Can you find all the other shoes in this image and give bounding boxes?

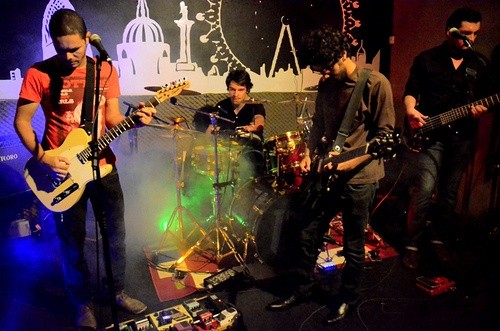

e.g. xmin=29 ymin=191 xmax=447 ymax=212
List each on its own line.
xmin=435 ymin=242 xmax=451 ymax=262
xmin=403 ymin=256 xmax=418 ymax=269
xmin=115 ymin=290 xmax=147 ymax=314
xmin=76 ymin=304 xmax=97 ymax=329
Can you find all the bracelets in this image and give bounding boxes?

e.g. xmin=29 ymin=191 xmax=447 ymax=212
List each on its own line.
xmin=39 ymin=153 xmax=45 ymax=162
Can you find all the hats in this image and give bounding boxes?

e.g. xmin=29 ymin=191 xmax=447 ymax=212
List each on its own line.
xmin=307 ymin=23 xmax=343 ymax=71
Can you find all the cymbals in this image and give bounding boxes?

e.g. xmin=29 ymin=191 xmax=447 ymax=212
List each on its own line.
xmin=278 ymin=98 xmax=316 ymax=103
xmin=144 ymin=87 xmax=201 ymax=95
xmin=145 ymin=124 xmax=201 ymax=133
xmin=236 ymin=99 xmax=272 ymax=104
xmin=158 ymin=134 xmax=192 ymax=138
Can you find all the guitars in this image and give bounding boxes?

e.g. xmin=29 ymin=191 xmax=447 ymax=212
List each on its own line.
xmin=24 ymin=77 xmax=191 ymax=212
xmin=301 ymin=132 xmax=407 ymax=212
xmin=402 ymin=94 xmax=500 ymax=153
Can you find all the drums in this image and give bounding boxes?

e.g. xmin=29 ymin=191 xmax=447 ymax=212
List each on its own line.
xmin=191 ymin=145 xmax=239 ymax=176
xmin=261 ymin=131 xmax=310 ymax=175
xmin=266 ymin=162 xmax=310 ymax=197
xmin=235 ymin=133 xmax=266 ymax=179
xmin=228 ymin=176 xmax=301 ymax=268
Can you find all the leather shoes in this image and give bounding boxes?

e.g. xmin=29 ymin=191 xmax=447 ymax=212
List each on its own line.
xmin=268 ymin=289 xmax=313 ymax=312
xmin=325 ymin=292 xmax=361 ymax=324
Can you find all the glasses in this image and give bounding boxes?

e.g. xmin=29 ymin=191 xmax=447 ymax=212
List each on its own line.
xmin=318 ymin=59 xmax=338 ymax=72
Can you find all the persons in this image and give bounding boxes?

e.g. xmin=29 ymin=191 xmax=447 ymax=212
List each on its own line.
xmin=14 ymin=9 xmax=157 ymax=329
xmin=267 ymin=25 xmax=395 ymax=312
xmin=403 ymin=7 xmax=500 ymax=269
xmin=208 ymin=70 xmax=266 ymax=176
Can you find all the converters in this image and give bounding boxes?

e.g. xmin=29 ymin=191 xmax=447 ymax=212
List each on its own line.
xmin=204 ymin=268 xmax=240 ymax=292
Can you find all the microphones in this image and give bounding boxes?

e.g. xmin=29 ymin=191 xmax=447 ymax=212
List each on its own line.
xmin=296 ymin=97 xmax=307 ymax=125
xmin=90 ymin=34 xmax=112 ymax=66
xmin=449 ymin=27 xmax=470 ymax=42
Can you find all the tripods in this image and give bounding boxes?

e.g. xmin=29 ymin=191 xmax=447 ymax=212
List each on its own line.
xmin=138 ymin=98 xmax=257 ymax=286
